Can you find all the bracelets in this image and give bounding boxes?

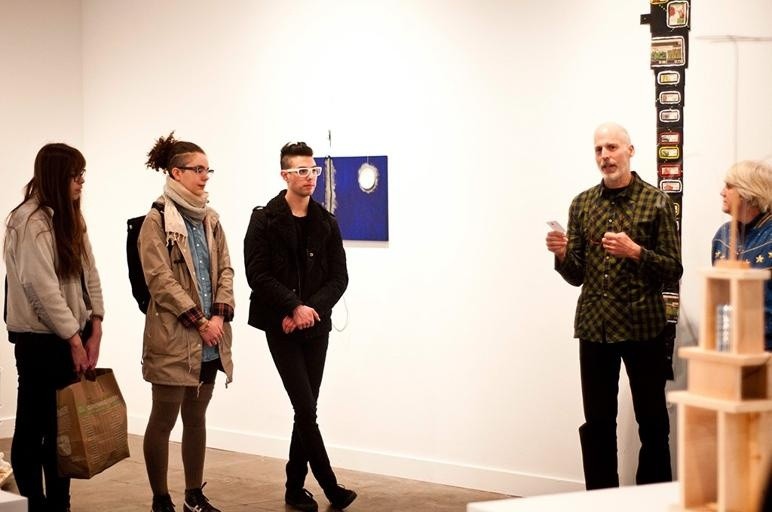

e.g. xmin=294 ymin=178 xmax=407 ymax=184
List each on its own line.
xmin=194 ymin=316 xmax=211 ymax=332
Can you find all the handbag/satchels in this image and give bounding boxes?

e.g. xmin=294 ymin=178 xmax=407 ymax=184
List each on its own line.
xmin=127 ymin=202 xmax=174 ymax=312
xmin=56 ymin=366 xmax=129 ymax=479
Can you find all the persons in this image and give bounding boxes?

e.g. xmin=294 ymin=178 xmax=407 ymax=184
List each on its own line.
xmin=3 ymin=142 xmax=104 ymax=512
xmin=137 ymin=129 xmax=235 ymax=512
xmin=546 ymin=121 xmax=684 ymax=490
xmin=711 ymin=161 xmax=772 ymax=353
xmin=244 ymin=142 xmax=357 ymax=512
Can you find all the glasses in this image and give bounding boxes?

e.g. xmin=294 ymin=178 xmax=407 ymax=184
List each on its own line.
xmin=72 ymin=169 xmax=86 ymax=183
xmin=288 ymin=167 xmax=322 ymax=177
xmin=177 ymin=165 xmax=214 ymax=178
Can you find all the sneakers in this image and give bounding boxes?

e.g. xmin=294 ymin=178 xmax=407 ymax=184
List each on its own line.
xmin=327 ymin=488 xmax=356 ymax=510
xmin=285 ymin=487 xmax=317 ymax=511
xmin=183 ymin=491 xmax=221 ymax=511
xmin=151 ymin=495 xmax=175 ymax=512
